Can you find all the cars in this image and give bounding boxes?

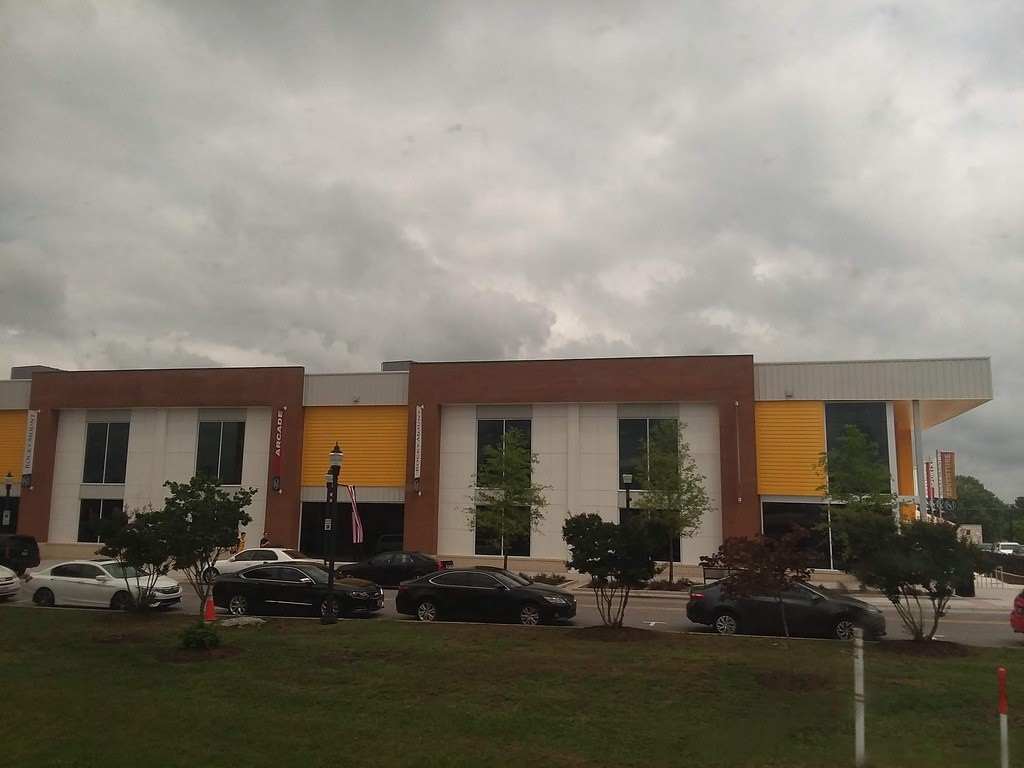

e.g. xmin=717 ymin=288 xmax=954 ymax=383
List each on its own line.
xmin=198 ymin=547 xmax=383 ymax=618
xmin=0 ymin=533 xmax=183 ymax=612
xmin=976 ymin=542 xmax=1024 ymax=559
xmin=395 ymin=566 xmax=577 ymax=624
xmin=336 ymin=550 xmax=454 ymax=589
xmin=685 ymin=570 xmax=888 ymax=642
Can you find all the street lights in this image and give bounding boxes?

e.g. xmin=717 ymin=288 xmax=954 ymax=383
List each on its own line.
xmin=622 ymin=472 xmax=632 ymax=520
xmin=4 ymin=469 xmax=12 ymax=534
xmin=321 ymin=441 xmax=345 ymax=622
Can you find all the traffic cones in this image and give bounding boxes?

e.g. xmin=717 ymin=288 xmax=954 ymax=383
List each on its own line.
xmin=203 ymin=582 xmax=217 ymax=621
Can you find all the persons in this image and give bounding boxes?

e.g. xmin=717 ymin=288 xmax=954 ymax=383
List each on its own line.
xmin=235 ymin=532 xmax=246 ymax=553
xmin=260 ymin=532 xmax=270 ymax=548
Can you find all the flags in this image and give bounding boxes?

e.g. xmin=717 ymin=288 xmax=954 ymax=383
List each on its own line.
xmin=347 ymin=485 xmax=363 ymax=543
